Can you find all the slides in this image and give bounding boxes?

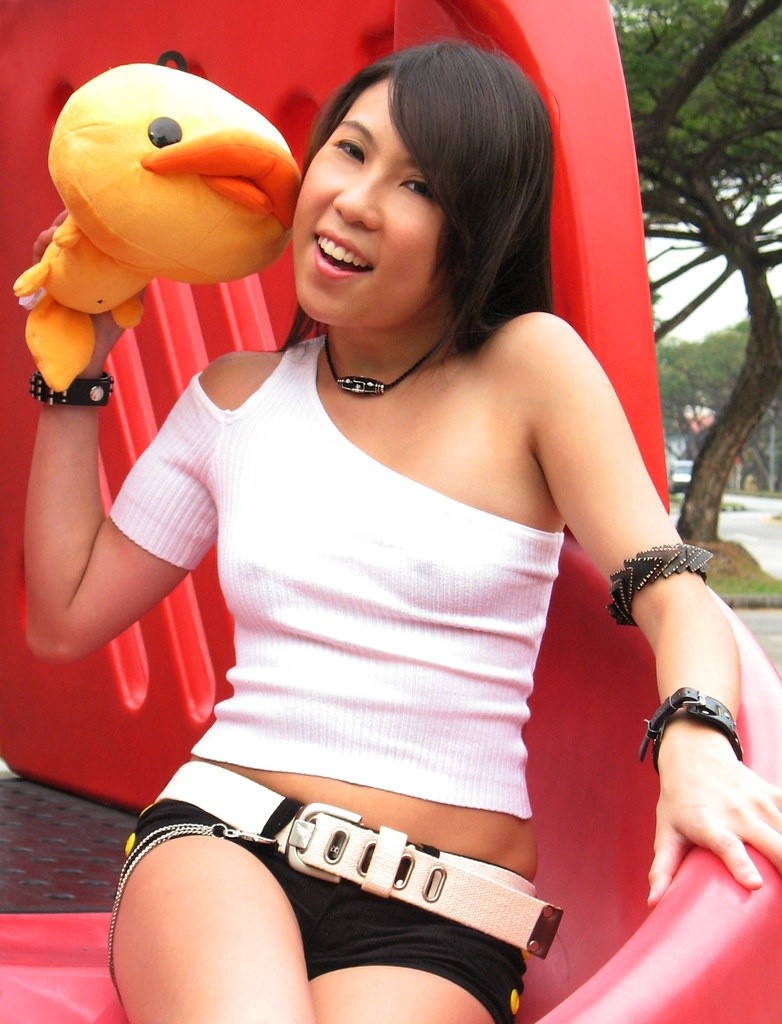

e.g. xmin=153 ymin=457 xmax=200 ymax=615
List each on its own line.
xmin=0 ymin=1 xmax=782 ymax=1024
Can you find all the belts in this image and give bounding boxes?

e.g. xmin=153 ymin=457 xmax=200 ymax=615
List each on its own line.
xmin=156 ymin=761 xmax=564 ymax=961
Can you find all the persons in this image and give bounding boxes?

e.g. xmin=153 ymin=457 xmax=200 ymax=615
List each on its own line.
xmin=25 ymin=42 xmax=780 ymax=1023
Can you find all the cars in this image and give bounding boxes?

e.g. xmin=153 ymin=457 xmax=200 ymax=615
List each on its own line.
xmin=669 ymin=460 xmax=693 ymax=493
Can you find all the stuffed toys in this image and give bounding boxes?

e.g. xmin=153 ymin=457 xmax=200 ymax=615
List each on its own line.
xmin=12 ymin=50 xmax=303 ymax=395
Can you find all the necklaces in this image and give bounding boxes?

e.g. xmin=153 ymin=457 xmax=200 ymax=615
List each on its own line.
xmin=324 ymin=317 xmax=453 ymax=397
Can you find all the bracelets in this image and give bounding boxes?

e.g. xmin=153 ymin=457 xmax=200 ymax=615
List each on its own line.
xmin=28 ymin=370 xmax=114 ymax=407
xmin=637 ymin=687 xmax=743 ymax=776
xmin=605 ymin=543 xmax=715 ymax=627
